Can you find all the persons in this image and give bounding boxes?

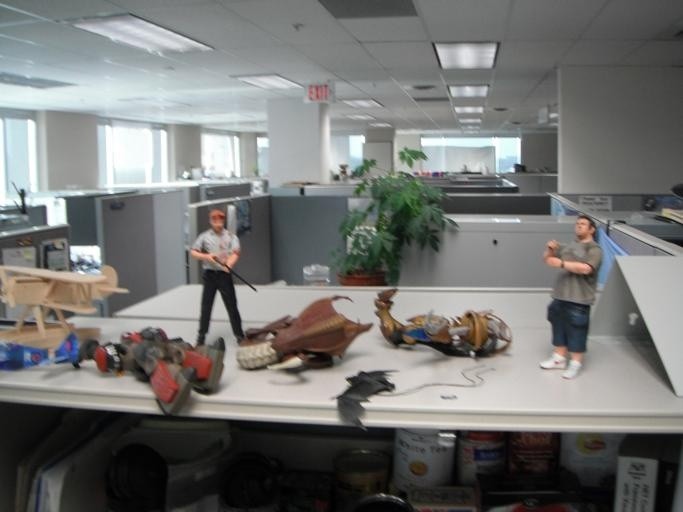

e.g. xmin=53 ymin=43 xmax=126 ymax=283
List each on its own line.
xmin=190 ymin=209 xmax=247 ymax=350
xmin=539 ymin=216 xmax=602 ymax=380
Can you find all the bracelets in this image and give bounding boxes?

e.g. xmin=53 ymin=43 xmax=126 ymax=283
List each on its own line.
xmin=560 ymin=260 xmax=564 ymax=269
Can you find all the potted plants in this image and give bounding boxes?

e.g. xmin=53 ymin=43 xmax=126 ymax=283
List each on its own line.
xmin=332 ymin=147 xmax=460 ymax=286
